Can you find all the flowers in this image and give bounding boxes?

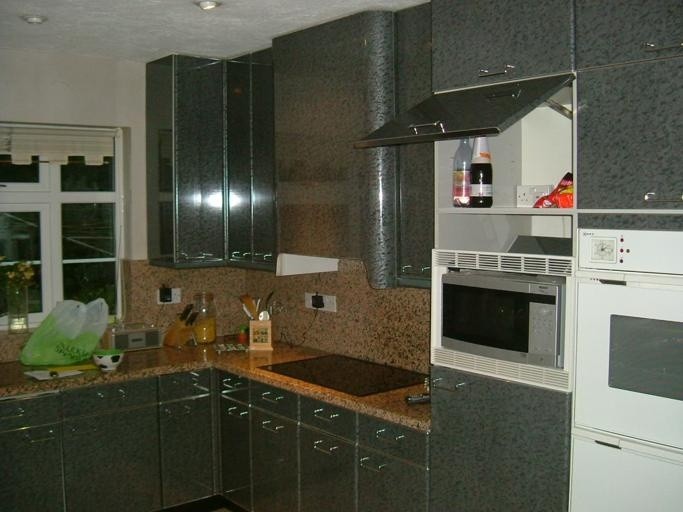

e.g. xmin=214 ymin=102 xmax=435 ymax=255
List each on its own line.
xmin=3 ymin=261 xmax=35 ymax=323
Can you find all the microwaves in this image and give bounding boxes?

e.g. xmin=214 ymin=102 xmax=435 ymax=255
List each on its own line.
xmin=439 ymin=267 xmax=567 ymax=370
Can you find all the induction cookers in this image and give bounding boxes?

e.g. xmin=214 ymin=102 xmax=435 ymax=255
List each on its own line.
xmin=256 ymin=354 xmax=430 ymax=397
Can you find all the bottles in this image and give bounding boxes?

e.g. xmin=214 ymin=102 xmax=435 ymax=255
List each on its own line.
xmin=191 ymin=291 xmax=216 ymax=343
xmin=452 ymin=135 xmax=493 ymax=208
xmin=237 ymin=324 xmax=249 ymax=346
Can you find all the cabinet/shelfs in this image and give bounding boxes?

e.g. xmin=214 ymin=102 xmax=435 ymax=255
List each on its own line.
xmin=162 ymin=369 xmax=215 ymax=512
xmin=220 ymin=371 xmax=428 ymax=511
xmin=0 ymin=378 xmax=160 ymax=512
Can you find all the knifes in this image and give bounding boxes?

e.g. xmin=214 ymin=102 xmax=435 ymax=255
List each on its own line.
xmin=179 ymin=304 xmax=200 ymax=327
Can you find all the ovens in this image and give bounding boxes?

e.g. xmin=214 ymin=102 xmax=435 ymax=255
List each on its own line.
xmin=568 ymin=228 xmax=683 ymax=512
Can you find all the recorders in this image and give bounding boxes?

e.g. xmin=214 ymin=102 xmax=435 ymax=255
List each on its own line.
xmin=111 ymin=321 xmax=161 ymax=353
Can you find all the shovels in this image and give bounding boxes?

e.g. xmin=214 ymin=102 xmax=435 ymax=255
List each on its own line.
xmin=240 ymin=290 xmax=273 ymax=320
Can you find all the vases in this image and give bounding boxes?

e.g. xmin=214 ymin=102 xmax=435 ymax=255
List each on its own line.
xmin=9 ymin=286 xmax=27 ymax=330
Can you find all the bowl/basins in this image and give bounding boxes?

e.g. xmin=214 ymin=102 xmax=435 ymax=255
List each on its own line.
xmin=93 ymin=349 xmax=124 ymax=372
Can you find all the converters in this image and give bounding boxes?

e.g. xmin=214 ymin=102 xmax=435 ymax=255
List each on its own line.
xmin=160 ymin=288 xmax=171 ymax=302
xmin=312 ymin=296 xmax=323 ymax=308
